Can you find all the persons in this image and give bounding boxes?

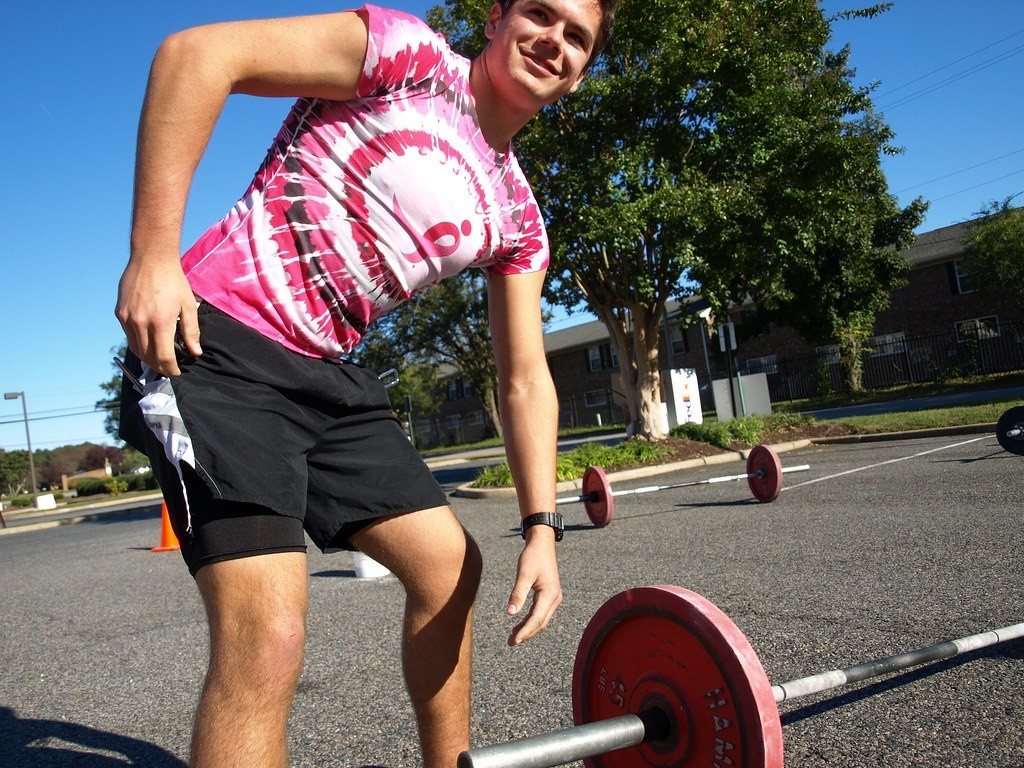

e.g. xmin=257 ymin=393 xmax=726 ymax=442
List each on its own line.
xmin=115 ymin=0 xmax=617 ymax=767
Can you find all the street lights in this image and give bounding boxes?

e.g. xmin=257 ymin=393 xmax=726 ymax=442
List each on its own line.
xmin=4 ymin=391 xmax=38 ymax=507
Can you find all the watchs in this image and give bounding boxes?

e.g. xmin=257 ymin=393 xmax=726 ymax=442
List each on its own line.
xmin=520 ymin=512 xmax=565 ymax=541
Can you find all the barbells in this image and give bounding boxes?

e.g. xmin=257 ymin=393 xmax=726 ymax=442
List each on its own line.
xmin=454 ymin=585 xmax=1024 ymax=767
xmin=555 ymin=444 xmax=811 ymax=528
xmin=996 ymin=406 xmax=1024 ymax=457
